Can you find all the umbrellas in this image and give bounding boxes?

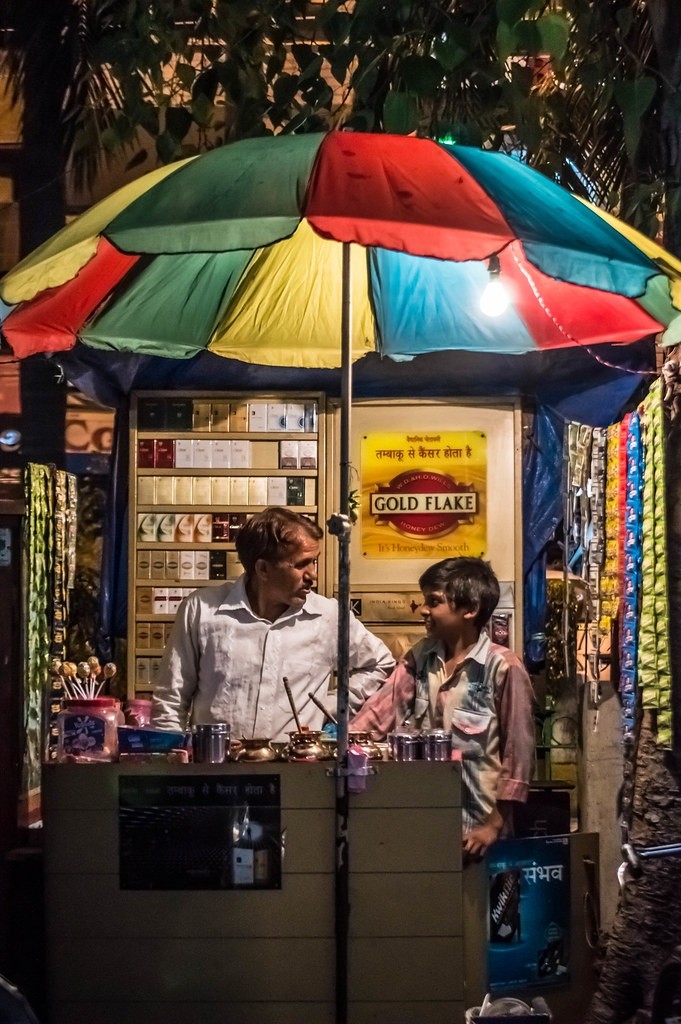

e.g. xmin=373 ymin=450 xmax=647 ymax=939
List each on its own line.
xmin=0 ymin=131 xmax=680 ymax=1024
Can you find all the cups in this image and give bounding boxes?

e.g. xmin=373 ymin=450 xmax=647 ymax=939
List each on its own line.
xmin=388 ymin=730 xmax=453 ymax=761
xmin=192 ymin=723 xmax=230 ymax=764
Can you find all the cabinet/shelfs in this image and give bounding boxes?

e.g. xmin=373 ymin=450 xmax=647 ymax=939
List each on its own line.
xmin=125 ymin=390 xmax=325 ymax=699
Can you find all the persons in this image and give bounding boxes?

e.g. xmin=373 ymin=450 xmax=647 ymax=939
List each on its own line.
xmin=349 ymin=557 xmax=534 ymax=863
xmin=149 ymin=505 xmax=394 ymax=749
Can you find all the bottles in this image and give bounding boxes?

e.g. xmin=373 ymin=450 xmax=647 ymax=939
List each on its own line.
xmin=122 ymin=699 xmax=151 ymax=727
xmin=57 ymin=698 xmax=125 ymax=763
